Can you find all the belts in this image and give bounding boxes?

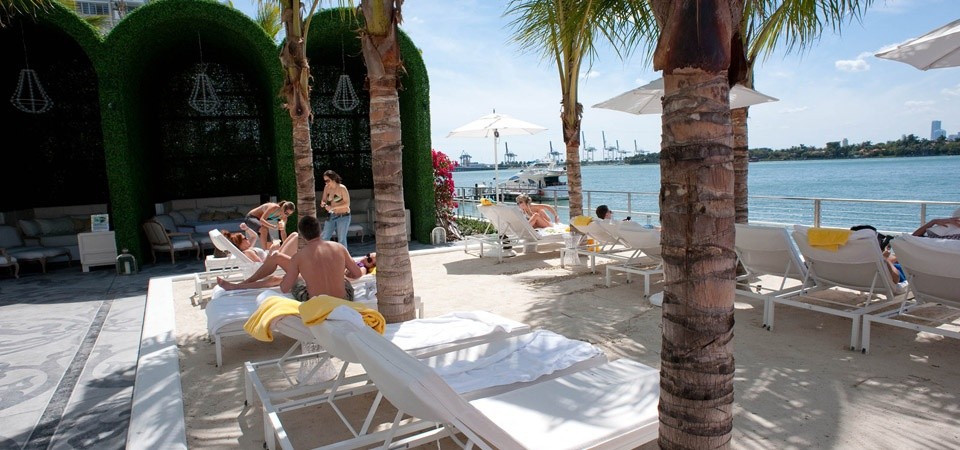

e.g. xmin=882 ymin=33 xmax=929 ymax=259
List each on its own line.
xmin=326 ymin=213 xmax=350 ymax=221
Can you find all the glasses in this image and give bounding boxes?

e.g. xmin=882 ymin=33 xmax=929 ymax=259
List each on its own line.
xmin=608 ymin=209 xmax=612 ymax=214
xmin=241 ymin=236 xmax=249 ymax=241
xmin=284 ymin=208 xmax=291 ymax=216
xmin=516 ymin=201 xmax=524 ymax=206
xmin=366 ymin=253 xmax=374 ymax=265
xmin=324 ymin=179 xmax=331 ymax=183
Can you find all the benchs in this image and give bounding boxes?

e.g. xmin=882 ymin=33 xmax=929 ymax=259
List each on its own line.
xmin=315 ymin=186 xmax=412 ymax=242
xmin=155 ymin=191 xmax=263 ymax=252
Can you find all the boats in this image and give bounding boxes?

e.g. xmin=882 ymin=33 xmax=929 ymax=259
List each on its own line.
xmin=494 ymin=162 xmax=570 ymax=200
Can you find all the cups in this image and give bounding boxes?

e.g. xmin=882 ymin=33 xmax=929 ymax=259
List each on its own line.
xmin=647 ymin=215 xmax=651 ymax=225
xmin=279 ymin=221 xmax=285 ymax=231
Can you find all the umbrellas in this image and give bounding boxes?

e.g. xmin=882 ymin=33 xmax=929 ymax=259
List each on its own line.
xmin=448 ymin=108 xmax=549 ymax=205
xmin=874 ymin=17 xmax=960 ymax=72
xmin=590 ymin=75 xmax=781 ymax=116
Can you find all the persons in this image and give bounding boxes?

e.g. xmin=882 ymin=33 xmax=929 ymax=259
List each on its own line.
xmin=595 ymin=204 xmax=661 ymax=230
xmin=849 ymin=209 xmax=960 ymax=285
xmin=209 ymin=164 xmax=378 ymax=313
xmin=515 ymin=192 xmax=559 ymax=230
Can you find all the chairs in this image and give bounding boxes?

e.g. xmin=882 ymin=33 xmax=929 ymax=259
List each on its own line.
xmin=478 ymin=201 xmax=540 ymax=258
xmin=259 ymin=298 xmax=622 ymax=448
xmin=1 ymin=203 xmax=110 ymax=279
xmin=497 ymin=201 xmax=572 ymax=257
xmin=193 ymin=224 xmax=432 ymax=368
xmin=573 ymin=212 xmax=958 ymax=361
xmin=239 ymin=296 xmax=534 ymax=450
xmin=143 ymin=220 xmax=201 ymax=264
xmin=338 ymin=318 xmax=659 ymax=449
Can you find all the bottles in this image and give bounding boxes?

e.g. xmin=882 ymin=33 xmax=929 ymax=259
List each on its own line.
xmin=324 ymin=204 xmax=335 ymax=215
